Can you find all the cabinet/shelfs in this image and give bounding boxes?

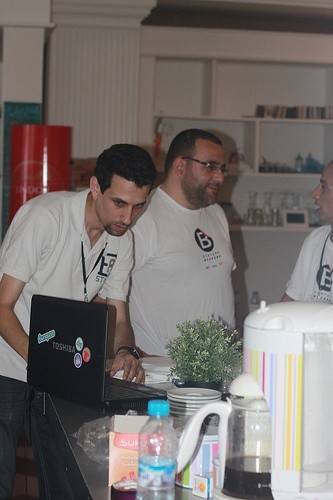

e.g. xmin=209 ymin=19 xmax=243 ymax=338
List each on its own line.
xmin=142 ymin=26 xmax=333 ymax=231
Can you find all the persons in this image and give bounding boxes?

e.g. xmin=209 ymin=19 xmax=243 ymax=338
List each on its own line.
xmin=280 ymin=160 xmax=333 ymax=305
xmin=0 ymin=143 xmax=157 ymax=500
xmin=90 ymin=127 xmax=237 ymax=355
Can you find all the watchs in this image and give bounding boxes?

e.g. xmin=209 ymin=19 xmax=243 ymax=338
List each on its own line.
xmin=114 ymin=346 xmax=140 ymax=358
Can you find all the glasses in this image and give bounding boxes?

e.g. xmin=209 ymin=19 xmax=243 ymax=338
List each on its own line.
xmin=183 ymin=157 xmax=228 ymax=176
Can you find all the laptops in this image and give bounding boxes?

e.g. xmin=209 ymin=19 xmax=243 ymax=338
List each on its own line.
xmin=28 ymin=294 xmax=168 ymax=411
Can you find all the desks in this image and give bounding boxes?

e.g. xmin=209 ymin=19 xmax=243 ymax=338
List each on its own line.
xmin=29 ymin=386 xmax=146 ymax=500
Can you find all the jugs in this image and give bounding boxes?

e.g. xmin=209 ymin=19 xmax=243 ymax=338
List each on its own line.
xmin=175 ymin=374 xmax=274 ymax=500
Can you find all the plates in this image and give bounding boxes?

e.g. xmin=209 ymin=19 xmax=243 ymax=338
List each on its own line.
xmin=166 ymin=388 xmax=222 ymax=415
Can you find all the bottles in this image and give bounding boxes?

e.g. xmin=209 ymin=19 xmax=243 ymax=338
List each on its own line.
xmin=259 ymin=192 xmax=274 ymax=226
xmin=277 ymin=193 xmax=285 ymax=225
xmin=136 ymin=400 xmax=176 ymax=500
xmin=296 ymin=154 xmax=303 ymax=173
xmin=244 ymin=191 xmax=258 ymax=226
xmin=251 ymin=291 xmax=260 ymax=311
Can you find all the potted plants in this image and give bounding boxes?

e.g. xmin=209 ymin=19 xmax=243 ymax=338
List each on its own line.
xmin=165 ymin=315 xmax=243 ymax=391
